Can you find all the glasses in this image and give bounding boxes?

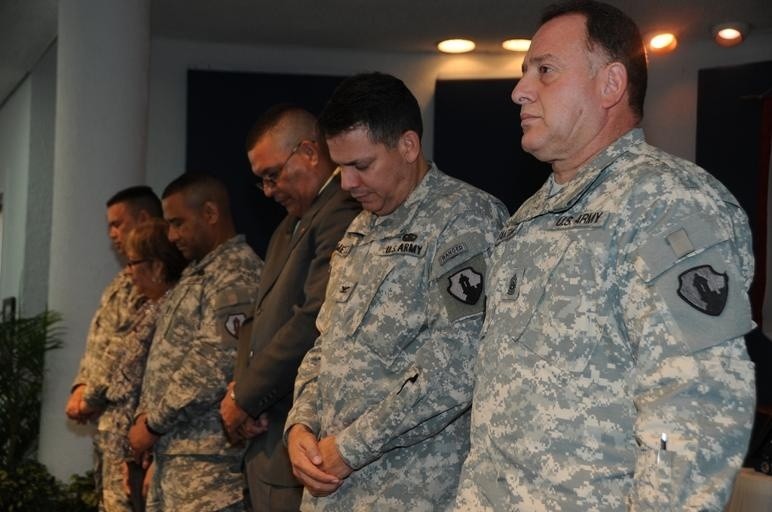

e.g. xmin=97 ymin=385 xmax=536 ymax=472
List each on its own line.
xmin=257 ymin=140 xmax=316 ymax=190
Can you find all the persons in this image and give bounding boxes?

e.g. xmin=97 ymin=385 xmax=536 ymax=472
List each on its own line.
xmin=284 ymin=68 xmax=509 ymax=512
xmin=65 ymin=186 xmax=191 ymax=512
xmin=452 ymin=6 xmax=757 ymax=512
xmin=126 ymin=170 xmax=265 ymax=512
xmin=217 ymin=105 xmax=362 ymax=512
